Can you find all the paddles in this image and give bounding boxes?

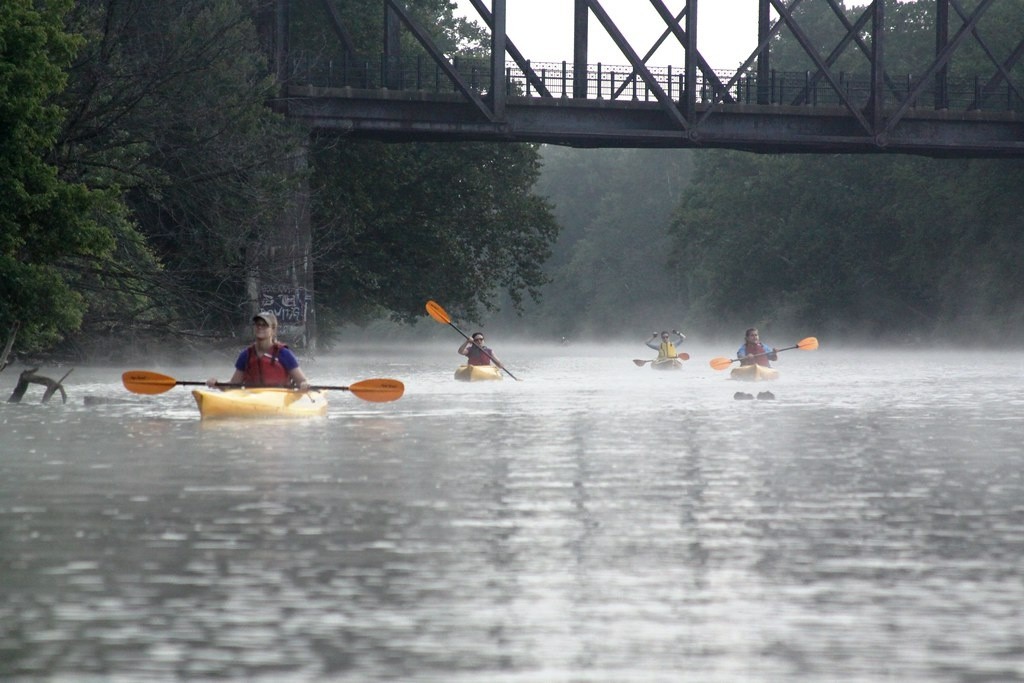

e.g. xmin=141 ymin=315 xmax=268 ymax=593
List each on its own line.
xmin=633 ymin=352 xmax=690 ymax=367
xmin=710 ymin=336 xmax=818 ymax=371
xmin=425 ymin=299 xmax=519 ymax=380
xmin=121 ymin=371 xmax=405 ymax=403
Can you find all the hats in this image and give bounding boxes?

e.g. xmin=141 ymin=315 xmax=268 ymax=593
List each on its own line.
xmin=252 ymin=312 xmax=277 ymax=328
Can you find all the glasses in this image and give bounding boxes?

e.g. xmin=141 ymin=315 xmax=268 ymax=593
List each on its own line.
xmin=474 ymin=337 xmax=484 ymax=342
xmin=255 ymin=323 xmax=269 ymax=330
xmin=662 ymin=336 xmax=668 ymax=339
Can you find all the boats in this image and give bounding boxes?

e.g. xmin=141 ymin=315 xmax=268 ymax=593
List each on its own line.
xmin=730 ymin=364 xmax=778 ymax=382
xmin=454 ymin=363 xmax=505 ymax=382
xmin=650 ymin=357 xmax=684 ymax=370
xmin=189 ymin=387 xmax=330 ymax=419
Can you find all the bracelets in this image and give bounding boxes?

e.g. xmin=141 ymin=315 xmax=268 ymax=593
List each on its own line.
xmin=678 ymin=332 xmax=680 ymax=335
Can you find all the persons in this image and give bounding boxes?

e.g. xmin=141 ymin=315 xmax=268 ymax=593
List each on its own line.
xmin=205 ymin=311 xmax=311 ymax=390
xmin=458 ymin=332 xmax=505 ymax=368
xmin=736 ymin=327 xmax=779 ymax=368
xmin=644 ymin=329 xmax=686 ymax=361
